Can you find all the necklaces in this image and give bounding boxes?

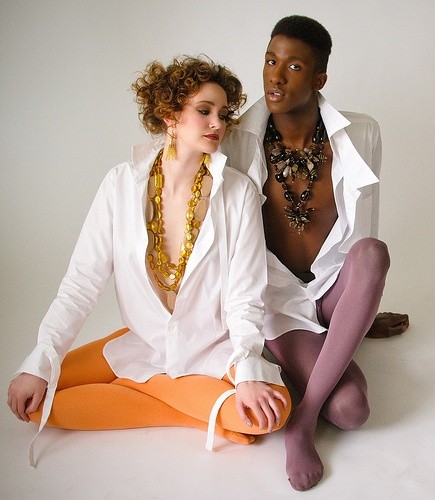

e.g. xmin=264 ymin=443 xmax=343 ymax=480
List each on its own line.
xmin=145 ymin=149 xmax=211 ymax=311
xmin=263 ymin=107 xmax=327 ymax=237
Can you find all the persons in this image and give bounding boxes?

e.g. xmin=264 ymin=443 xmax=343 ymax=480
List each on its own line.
xmin=6 ymin=54 xmax=292 ymax=468
xmin=216 ymin=15 xmax=411 ymax=492
